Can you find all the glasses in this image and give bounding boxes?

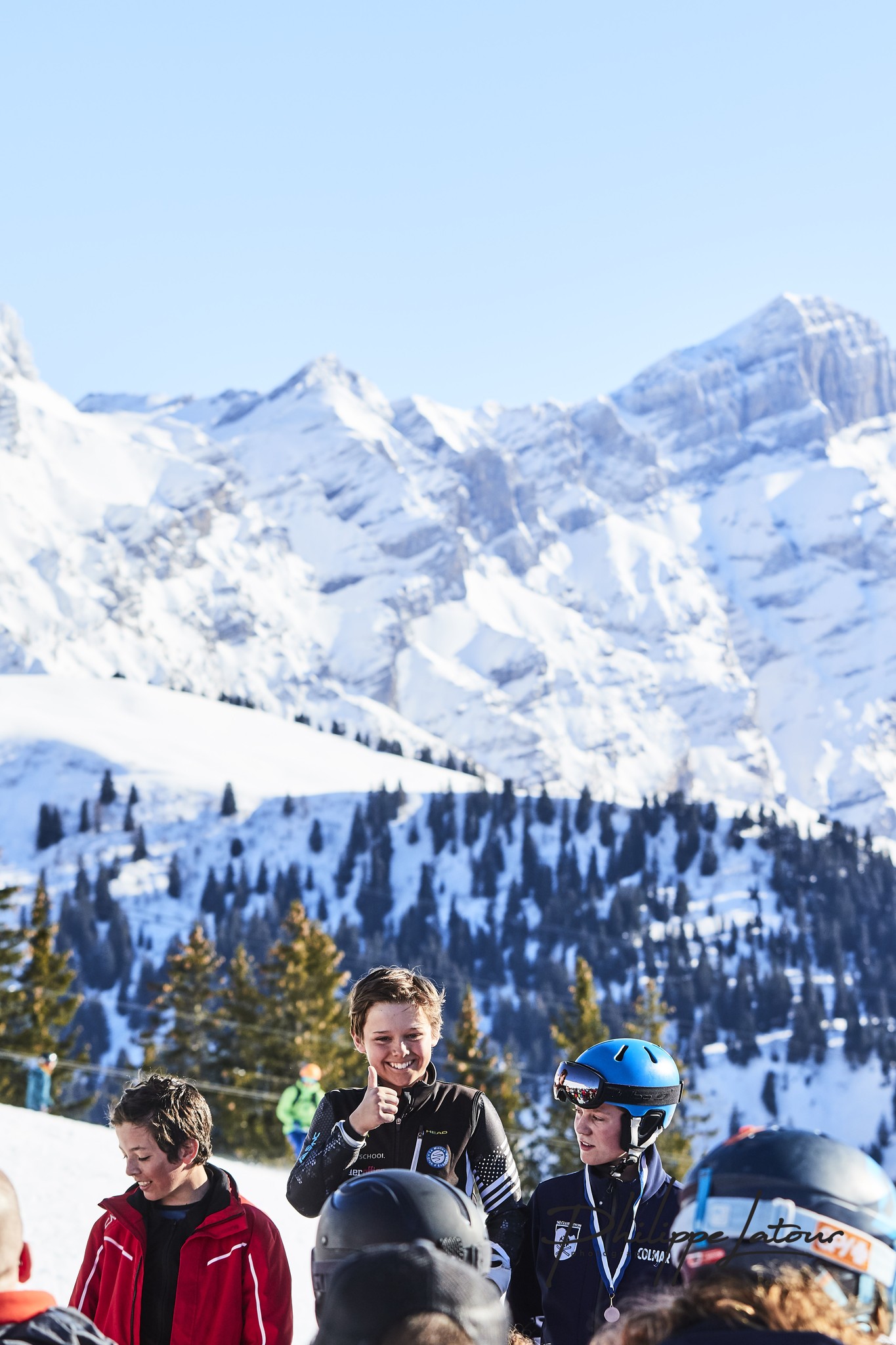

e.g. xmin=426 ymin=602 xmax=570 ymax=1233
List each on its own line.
xmin=553 ymin=1059 xmax=609 ymax=1110
xmin=301 ymin=1077 xmax=318 ymax=1084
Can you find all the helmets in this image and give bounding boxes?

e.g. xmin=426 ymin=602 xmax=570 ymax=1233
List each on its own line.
xmin=666 ymin=1123 xmax=896 ymax=1321
xmin=312 ymin=1168 xmax=487 ymax=1317
xmin=298 ymin=1063 xmax=321 ymax=1082
xmin=573 ymin=1036 xmax=681 ymax=1148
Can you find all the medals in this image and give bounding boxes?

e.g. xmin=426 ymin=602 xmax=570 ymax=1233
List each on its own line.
xmin=603 ymin=1305 xmax=620 ymax=1322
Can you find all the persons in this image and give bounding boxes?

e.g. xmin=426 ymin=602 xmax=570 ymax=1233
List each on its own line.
xmin=25 ymin=1050 xmax=60 ymax=1115
xmin=287 ymin=965 xmax=540 ymax=1300
xmin=310 ymin=1242 xmax=513 ymax=1345
xmin=591 ymin=1127 xmax=895 ymax=1345
xmin=509 ymin=1035 xmax=692 ymax=1344
xmin=276 ymin=1061 xmax=326 ymax=1161
xmin=0 ymin=1166 xmax=121 ymax=1345
xmin=312 ymin=1166 xmax=494 ymax=1328
xmin=550 ymin=1067 xmax=597 ymax=1104
xmin=67 ymin=1070 xmax=294 ymax=1344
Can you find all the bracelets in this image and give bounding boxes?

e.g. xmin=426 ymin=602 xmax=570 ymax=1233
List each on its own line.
xmin=344 ymin=1117 xmax=368 ymax=1142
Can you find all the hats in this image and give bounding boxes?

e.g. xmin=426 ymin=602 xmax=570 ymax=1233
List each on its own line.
xmin=316 ymin=1244 xmax=514 ymax=1345
